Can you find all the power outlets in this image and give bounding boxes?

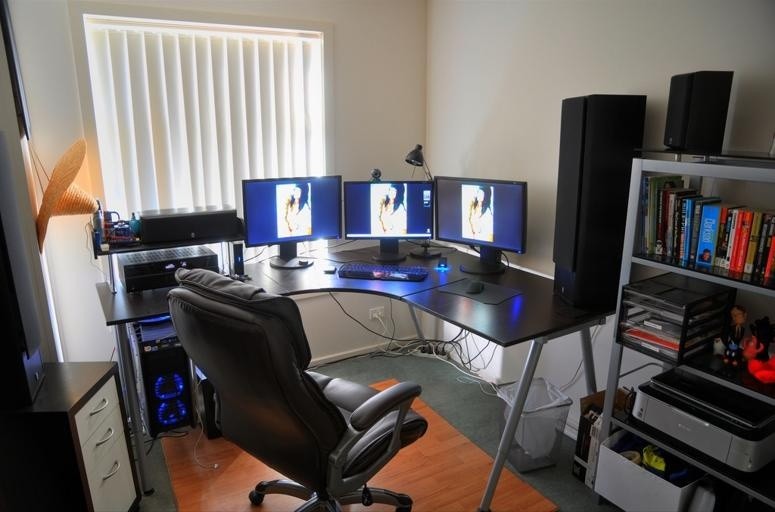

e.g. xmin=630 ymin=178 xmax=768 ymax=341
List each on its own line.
xmin=368 ymin=306 xmax=385 ymax=321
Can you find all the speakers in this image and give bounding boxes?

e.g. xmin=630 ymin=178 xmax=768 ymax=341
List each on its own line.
xmin=229 ymin=241 xmax=245 ymax=278
xmin=663 ymin=71 xmax=735 ymax=154
xmin=552 ymin=94 xmax=649 ymax=314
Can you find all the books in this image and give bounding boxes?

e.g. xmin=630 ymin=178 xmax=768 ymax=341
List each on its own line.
xmin=619 ymin=288 xmax=729 ymax=360
xmin=639 ymin=172 xmax=775 ymax=281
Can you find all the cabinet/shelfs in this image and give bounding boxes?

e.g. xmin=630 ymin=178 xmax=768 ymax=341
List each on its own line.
xmin=28 ymin=360 xmax=143 ymax=512
xmin=590 ymin=154 xmax=775 ymax=512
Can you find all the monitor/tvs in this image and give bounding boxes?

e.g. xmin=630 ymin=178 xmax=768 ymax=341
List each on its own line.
xmin=241 ymin=174 xmax=342 ymax=270
xmin=343 ymin=180 xmax=434 ymax=262
xmin=434 ymin=174 xmax=528 ymax=276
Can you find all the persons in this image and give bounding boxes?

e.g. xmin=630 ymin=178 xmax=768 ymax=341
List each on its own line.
xmin=467 ymin=186 xmax=493 ymax=241
xmin=280 ymin=181 xmax=311 ymax=235
xmin=375 ymin=183 xmax=408 ymax=234
xmin=712 ymin=301 xmax=775 ymax=384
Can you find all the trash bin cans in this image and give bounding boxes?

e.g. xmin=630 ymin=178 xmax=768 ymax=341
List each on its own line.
xmin=497 ymin=377 xmax=573 ymax=474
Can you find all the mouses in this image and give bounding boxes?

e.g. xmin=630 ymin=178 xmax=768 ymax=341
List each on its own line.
xmin=466 ymin=280 xmax=485 ymax=295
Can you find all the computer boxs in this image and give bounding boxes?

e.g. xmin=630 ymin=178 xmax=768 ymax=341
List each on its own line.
xmin=126 ymin=315 xmax=193 ymax=438
xmin=192 ymin=364 xmax=221 ymax=441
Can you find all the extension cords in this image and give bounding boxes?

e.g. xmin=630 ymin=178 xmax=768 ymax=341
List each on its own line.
xmin=408 ymin=349 xmax=449 ymax=361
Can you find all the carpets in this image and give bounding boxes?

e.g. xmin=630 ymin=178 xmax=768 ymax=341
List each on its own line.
xmin=159 ymin=378 xmax=562 ymax=512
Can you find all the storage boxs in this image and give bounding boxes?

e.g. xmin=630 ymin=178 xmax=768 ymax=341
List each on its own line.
xmin=593 ymin=428 xmax=712 ymax=512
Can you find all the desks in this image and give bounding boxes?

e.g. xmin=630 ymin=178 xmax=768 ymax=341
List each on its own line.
xmin=94 ymin=237 xmax=618 ymax=512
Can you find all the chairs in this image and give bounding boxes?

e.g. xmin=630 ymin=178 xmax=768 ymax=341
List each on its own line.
xmin=165 ymin=266 xmax=428 ymax=512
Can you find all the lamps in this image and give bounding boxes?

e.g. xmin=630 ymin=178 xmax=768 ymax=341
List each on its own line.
xmin=404 ymin=144 xmax=433 ymax=182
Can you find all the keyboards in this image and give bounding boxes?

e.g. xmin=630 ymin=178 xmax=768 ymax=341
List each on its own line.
xmin=337 ymin=261 xmax=429 ymax=283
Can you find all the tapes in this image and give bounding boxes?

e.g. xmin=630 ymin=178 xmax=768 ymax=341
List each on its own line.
xmin=620 ymin=451 xmax=641 ymax=464
